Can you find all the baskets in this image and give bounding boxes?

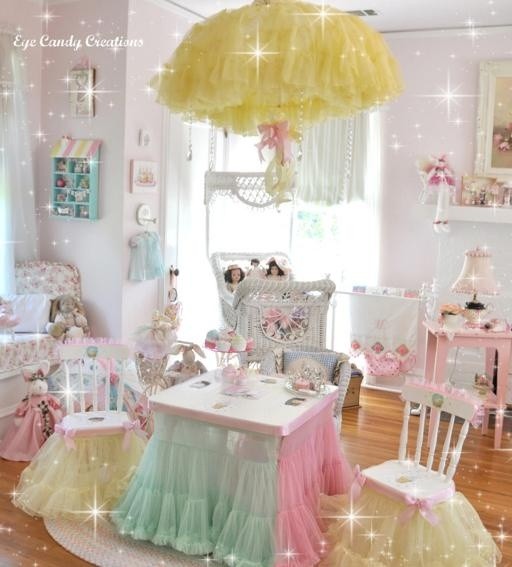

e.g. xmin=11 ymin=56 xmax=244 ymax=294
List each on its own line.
xmin=205 ymin=249 xmax=338 ymax=372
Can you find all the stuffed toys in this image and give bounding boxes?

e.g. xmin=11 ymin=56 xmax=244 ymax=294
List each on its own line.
xmin=416 ymin=153 xmax=459 ymax=233
xmin=166 ymin=342 xmax=208 ymax=384
xmin=0 ymin=359 xmax=65 ymax=461
xmin=47 ymin=292 xmax=88 ymax=337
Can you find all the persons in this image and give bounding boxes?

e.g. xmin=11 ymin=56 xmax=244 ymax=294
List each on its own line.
xmin=223 ymin=264 xmax=246 ymax=293
xmin=264 ymin=257 xmax=291 ymax=280
xmin=246 ymin=257 xmax=265 ymax=279
